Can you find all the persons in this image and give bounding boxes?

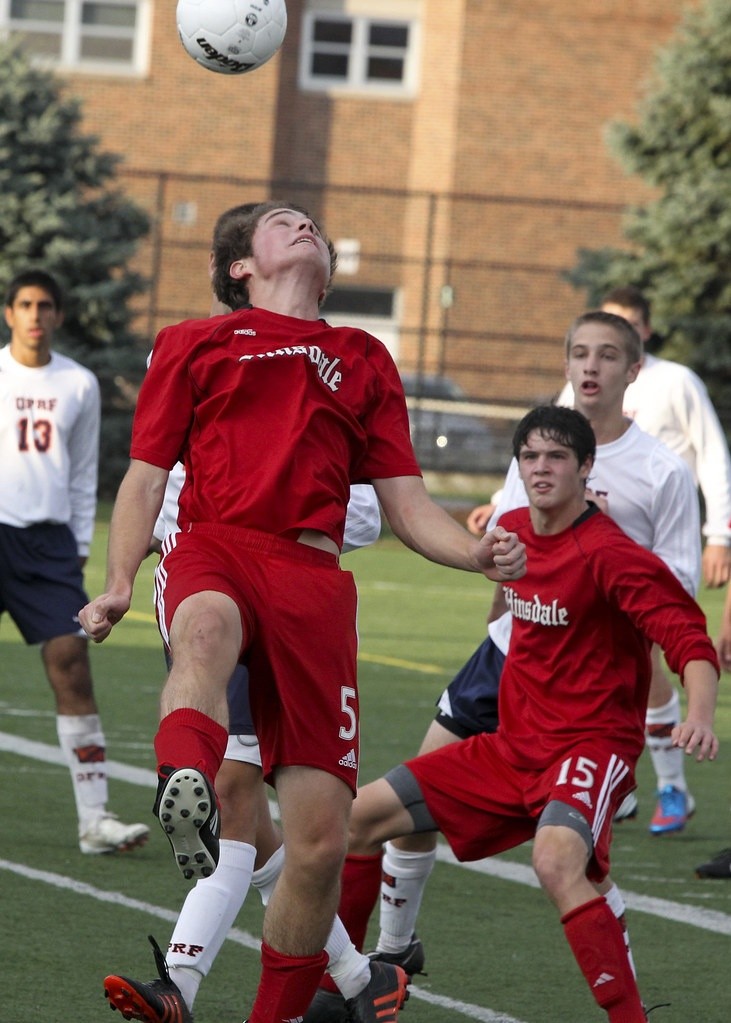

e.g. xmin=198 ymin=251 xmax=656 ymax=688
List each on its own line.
xmin=694 ymin=459 xmax=730 ymax=879
xmin=469 ymin=293 xmax=727 ymax=834
xmin=74 ymin=209 xmax=525 ymax=1022
xmin=103 ymin=203 xmax=383 ymax=1022
xmin=1 ymin=271 xmax=149 ymax=856
xmin=377 ymin=312 xmax=701 ymax=978
xmin=301 ymin=406 xmax=720 ymax=1023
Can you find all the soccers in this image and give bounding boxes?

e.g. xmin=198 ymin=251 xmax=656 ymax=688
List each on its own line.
xmin=177 ymin=0 xmax=287 ymax=74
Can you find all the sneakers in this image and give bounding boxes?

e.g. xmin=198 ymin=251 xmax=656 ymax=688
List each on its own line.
xmin=102 ymin=936 xmax=197 ymax=1023
xmin=152 ymin=765 xmax=223 ymax=881
xmin=647 ymin=784 xmax=696 ymax=834
xmin=302 ymin=990 xmax=346 ymax=1023
xmin=345 ymin=960 xmax=411 ymax=1023
xmin=367 ymin=931 xmax=428 ymax=977
xmin=611 ymin=792 xmax=639 ymax=824
xmin=78 ymin=811 xmax=150 ymax=855
xmin=694 ymin=847 xmax=731 ymax=879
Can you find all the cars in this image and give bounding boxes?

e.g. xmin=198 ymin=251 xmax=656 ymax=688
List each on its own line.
xmin=395 ymin=374 xmax=495 ymax=470
